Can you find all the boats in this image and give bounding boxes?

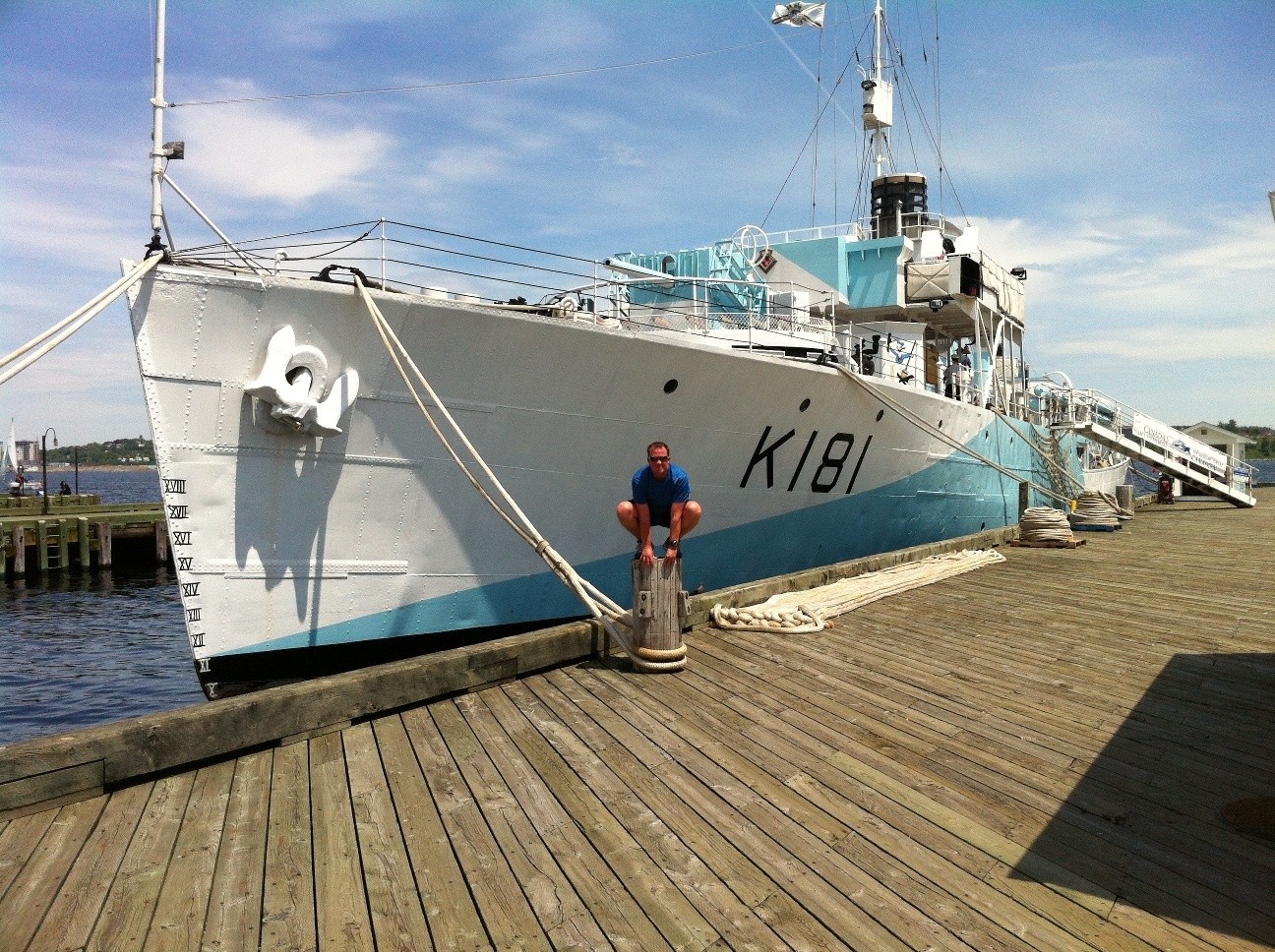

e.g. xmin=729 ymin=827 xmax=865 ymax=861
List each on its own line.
xmin=9 ymin=479 xmax=43 ymax=496
xmin=117 ymin=3 xmax=1132 ymax=700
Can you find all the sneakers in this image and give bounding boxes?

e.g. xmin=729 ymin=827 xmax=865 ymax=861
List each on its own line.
xmin=634 ymin=541 xmax=654 ymax=559
xmin=662 ymin=536 xmax=683 ymax=558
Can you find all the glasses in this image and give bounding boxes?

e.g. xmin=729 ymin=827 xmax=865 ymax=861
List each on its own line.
xmin=648 ymin=456 xmax=668 ymax=462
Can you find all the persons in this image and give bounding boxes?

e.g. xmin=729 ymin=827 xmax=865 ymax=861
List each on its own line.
xmin=59 ymin=481 xmax=72 ymax=495
xmin=617 ymin=442 xmax=702 ymax=561
xmin=851 ymin=349 xmax=868 ymax=377
xmin=955 ymin=348 xmax=971 ymax=378
xmin=942 ymin=354 xmax=963 ymax=401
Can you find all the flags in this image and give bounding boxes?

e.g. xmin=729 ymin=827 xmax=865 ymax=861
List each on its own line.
xmin=770 ymin=1 xmax=827 ymax=30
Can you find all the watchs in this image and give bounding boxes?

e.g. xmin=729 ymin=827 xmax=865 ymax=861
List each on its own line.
xmin=669 ymin=540 xmax=680 ymax=547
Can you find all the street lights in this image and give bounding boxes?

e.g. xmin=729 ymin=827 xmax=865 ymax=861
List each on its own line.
xmin=41 ymin=427 xmax=58 ymax=514
